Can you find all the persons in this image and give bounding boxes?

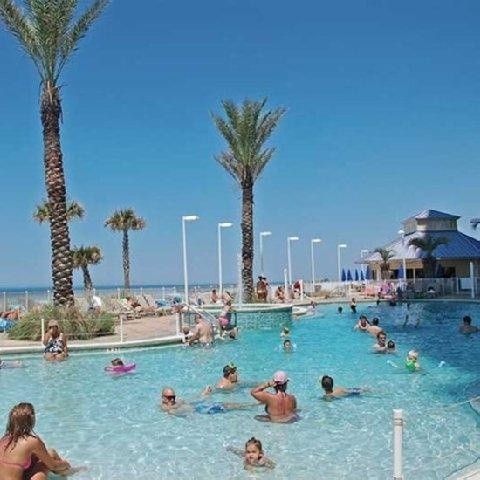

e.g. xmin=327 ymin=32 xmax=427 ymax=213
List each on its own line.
xmin=43 ymin=319 xmax=70 ymax=364
xmin=1 ymin=401 xmax=88 ymax=480
xmin=0 ymin=311 xmax=24 ymax=321
xmin=256 ymin=276 xmax=419 ymax=367
xmin=159 ymin=361 xmax=340 ymax=416
xmin=222 ymin=435 xmax=278 ymax=476
xmin=456 ymin=314 xmax=479 ymax=336
xmin=111 ymin=358 xmax=123 ymax=366
xmin=124 ymin=290 xmax=237 ymax=347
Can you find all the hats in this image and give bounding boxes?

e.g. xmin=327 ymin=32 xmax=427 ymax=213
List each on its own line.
xmin=48 ymin=319 xmax=59 ymax=328
xmin=223 ymin=365 xmax=237 ymax=377
xmin=272 ymin=371 xmax=291 ymax=387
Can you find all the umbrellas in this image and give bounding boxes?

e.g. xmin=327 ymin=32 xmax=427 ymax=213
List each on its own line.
xmin=397 ymin=263 xmax=404 ymax=297
xmin=354 ymin=267 xmax=360 ymax=282
xmin=347 ymin=268 xmax=353 ymax=291
xmin=366 ymin=267 xmax=372 ymax=280
xmin=341 ymin=267 xmax=347 ymax=282
xmin=360 ymin=270 xmax=366 ymax=281
xmin=435 ymin=263 xmax=447 ymax=297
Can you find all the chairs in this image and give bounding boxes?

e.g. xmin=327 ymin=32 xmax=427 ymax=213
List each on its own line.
xmin=112 ymin=296 xmax=173 ymax=321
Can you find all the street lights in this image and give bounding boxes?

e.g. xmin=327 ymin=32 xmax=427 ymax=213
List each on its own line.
xmin=181 ymin=214 xmax=200 ymax=306
xmin=338 ymin=243 xmax=348 ymax=282
xmin=287 ymin=236 xmax=300 ymax=284
xmin=311 ymin=238 xmax=324 ymax=284
xmin=360 ymin=249 xmax=368 ymax=266
xmin=259 ymin=230 xmax=272 ymax=273
xmin=398 ymin=229 xmax=407 ymax=283
xmin=218 ymin=222 xmax=234 ymax=298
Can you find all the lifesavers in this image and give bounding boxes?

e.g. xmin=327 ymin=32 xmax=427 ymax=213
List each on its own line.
xmin=106 ymin=362 xmax=136 ymax=375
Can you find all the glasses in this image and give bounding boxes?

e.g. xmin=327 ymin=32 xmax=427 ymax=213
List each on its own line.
xmin=164 ymin=395 xmax=176 ymax=401
